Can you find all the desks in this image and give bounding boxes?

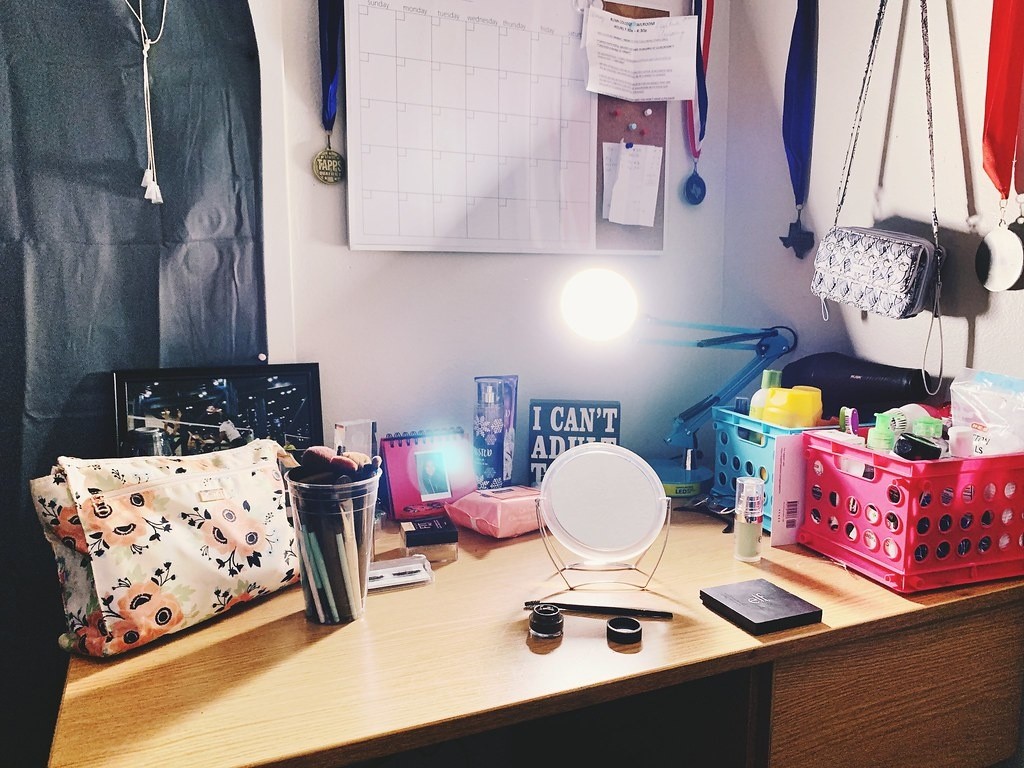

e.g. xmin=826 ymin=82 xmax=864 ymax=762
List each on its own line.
xmin=47 ymin=485 xmax=1024 ymax=768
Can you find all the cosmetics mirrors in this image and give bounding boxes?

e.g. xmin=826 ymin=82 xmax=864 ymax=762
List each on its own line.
xmin=537 ymin=442 xmax=667 ymax=564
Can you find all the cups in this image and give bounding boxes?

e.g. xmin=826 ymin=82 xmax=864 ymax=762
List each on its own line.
xmin=284 ymin=467 xmax=382 ymax=626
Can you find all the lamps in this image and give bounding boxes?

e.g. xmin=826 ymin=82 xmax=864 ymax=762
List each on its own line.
xmin=560 ymin=267 xmax=797 ymax=484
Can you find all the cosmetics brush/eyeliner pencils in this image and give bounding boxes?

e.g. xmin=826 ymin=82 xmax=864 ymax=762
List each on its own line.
xmin=291 ymin=445 xmax=382 ymax=624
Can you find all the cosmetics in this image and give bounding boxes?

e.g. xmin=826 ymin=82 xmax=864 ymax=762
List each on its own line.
xmin=473 ymin=375 xmax=518 ymax=490
xmin=366 ymin=515 xmax=459 ymax=591
xmin=728 ymin=369 xmax=991 ymax=564
xmin=529 ymin=605 xmax=564 ymax=639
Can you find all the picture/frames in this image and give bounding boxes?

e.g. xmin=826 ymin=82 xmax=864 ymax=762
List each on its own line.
xmin=112 ymin=363 xmax=324 ymax=523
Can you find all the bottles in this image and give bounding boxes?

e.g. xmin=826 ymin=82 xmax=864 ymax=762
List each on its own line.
xmin=749 ymin=368 xmax=782 ymax=443
xmin=940 ymin=426 xmax=978 ymax=503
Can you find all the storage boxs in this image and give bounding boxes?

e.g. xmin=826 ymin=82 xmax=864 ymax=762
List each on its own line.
xmin=334 ymin=419 xmax=378 ymax=459
xmin=711 ymin=406 xmax=876 ymax=533
xmin=797 ymin=426 xmax=1024 ymax=593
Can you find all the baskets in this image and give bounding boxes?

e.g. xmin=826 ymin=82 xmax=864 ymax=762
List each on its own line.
xmin=710 ymin=405 xmax=881 ymax=534
xmin=795 ymin=427 xmax=1024 ymax=592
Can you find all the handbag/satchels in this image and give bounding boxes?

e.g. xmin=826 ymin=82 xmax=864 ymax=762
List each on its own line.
xmin=810 ymin=227 xmax=944 ymax=323
xmin=29 ymin=437 xmax=304 ymax=658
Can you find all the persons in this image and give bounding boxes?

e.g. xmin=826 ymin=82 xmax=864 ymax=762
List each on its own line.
xmin=422 ymin=460 xmax=446 ymax=493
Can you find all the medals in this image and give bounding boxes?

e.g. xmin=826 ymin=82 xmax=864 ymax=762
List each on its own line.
xmin=313 ymin=147 xmax=345 ymax=185
xmin=685 ymin=172 xmax=706 ymax=205
xmin=974 ymin=221 xmax=1024 ymax=292
